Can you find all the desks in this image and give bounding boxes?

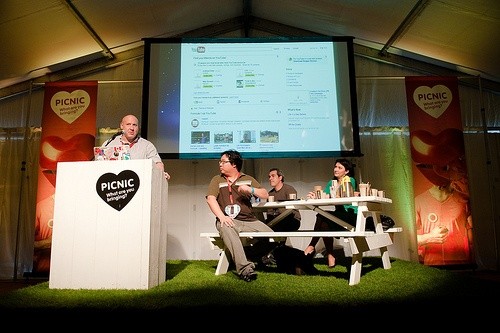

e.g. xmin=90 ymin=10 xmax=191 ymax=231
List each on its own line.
xmin=215 ymin=196 xmax=394 ymax=286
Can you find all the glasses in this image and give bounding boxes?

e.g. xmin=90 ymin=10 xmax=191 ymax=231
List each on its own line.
xmin=219 ymin=160 xmax=229 ymax=164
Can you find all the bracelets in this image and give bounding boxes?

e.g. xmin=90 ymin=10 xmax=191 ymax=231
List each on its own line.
xmin=251 ymin=187 xmax=254 ymax=194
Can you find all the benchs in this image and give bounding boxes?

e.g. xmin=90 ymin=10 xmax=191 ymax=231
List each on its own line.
xmin=200 ymin=227 xmax=402 ymax=237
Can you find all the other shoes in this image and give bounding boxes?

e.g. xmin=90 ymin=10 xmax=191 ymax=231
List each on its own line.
xmin=329 ymin=257 xmax=336 ymax=269
xmin=243 ymin=273 xmax=258 ymax=281
xmin=305 ymin=248 xmax=316 ymax=258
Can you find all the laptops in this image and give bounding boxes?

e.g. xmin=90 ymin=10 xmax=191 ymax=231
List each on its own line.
xmin=93 ymin=145 xmax=130 ymax=161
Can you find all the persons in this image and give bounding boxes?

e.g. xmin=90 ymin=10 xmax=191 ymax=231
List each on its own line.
xmin=415 ymin=186 xmax=472 ymax=266
xmin=205 ymin=151 xmax=274 ymax=281
xmin=35 ymin=190 xmax=55 ymax=276
xmin=100 ymin=114 xmax=170 ymax=180
xmin=267 ymin=168 xmax=301 ymax=231
xmin=304 ymin=160 xmax=357 ymax=268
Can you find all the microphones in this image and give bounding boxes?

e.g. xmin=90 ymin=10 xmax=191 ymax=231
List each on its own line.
xmin=111 ymin=130 xmax=125 ymax=138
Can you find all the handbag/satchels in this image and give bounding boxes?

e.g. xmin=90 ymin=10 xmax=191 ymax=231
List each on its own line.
xmin=365 ymin=215 xmax=395 ymax=232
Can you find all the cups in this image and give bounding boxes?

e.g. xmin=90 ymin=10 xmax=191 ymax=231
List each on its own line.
xmin=330 ymin=186 xmax=340 ymax=198
xmin=289 ymin=194 xmax=296 ymax=199
xmin=340 ymin=182 xmax=354 ymax=197
xmin=314 ymin=186 xmax=321 ymax=199
xmin=268 ymin=196 xmax=274 ymax=202
xmin=358 ymin=184 xmax=386 ymax=197
